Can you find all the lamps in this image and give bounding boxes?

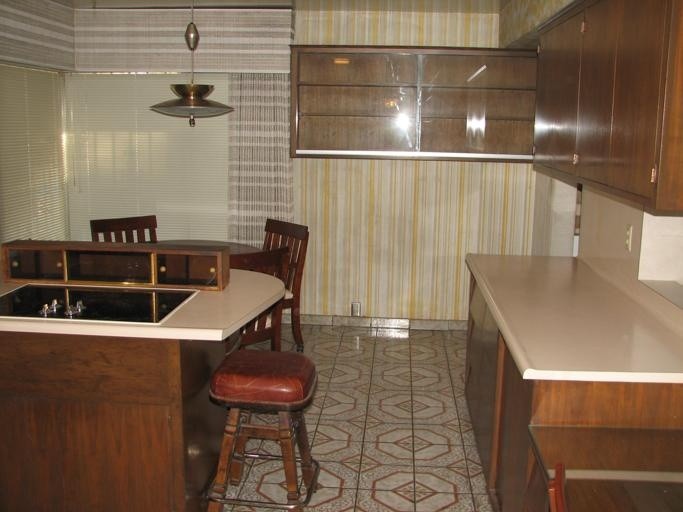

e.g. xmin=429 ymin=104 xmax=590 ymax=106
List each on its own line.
xmin=149 ymin=0 xmax=235 ymax=128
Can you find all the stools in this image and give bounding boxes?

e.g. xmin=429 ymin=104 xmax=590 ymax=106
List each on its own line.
xmin=206 ymin=348 xmax=320 ymax=509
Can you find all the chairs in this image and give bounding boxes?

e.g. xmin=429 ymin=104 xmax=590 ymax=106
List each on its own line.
xmin=88 ymin=214 xmax=310 ymax=353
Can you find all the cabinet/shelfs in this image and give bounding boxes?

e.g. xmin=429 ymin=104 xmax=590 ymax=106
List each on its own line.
xmin=531 ymin=0 xmax=683 ymax=217
xmin=287 ymin=42 xmax=539 ymax=164
